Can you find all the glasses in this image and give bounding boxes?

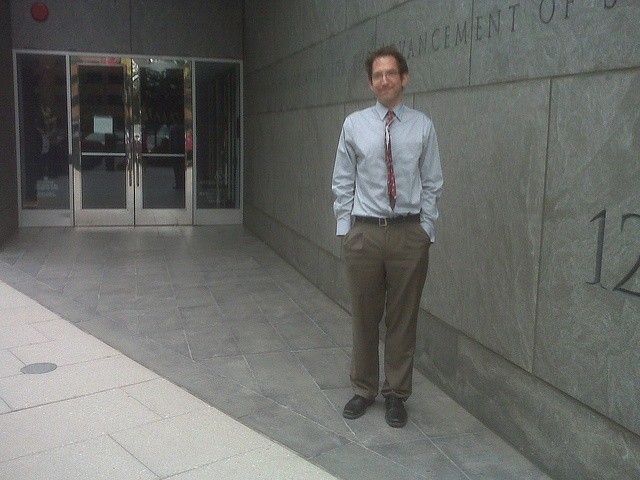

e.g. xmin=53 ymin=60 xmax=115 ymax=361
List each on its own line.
xmin=372 ymin=71 xmax=400 ymax=81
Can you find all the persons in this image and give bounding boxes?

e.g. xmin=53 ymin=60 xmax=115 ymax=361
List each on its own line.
xmin=331 ymin=45 xmax=445 ymax=429
xmin=168 ymin=116 xmax=185 ymax=188
xmin=125 ymin=134 xmax=134 ymax=187
xmin=23 ymin=104 xmax=59 ymax=208
xmin=134 ymin=133 xmax=141 ymax=185
xmin=185 ymin=128 xmax=194 ymax=159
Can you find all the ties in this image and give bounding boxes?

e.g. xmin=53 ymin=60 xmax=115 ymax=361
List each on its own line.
xmin=385 ymin=111 xmax=396 ymax=212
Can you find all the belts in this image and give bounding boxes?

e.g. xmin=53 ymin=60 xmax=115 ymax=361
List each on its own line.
xmin=355 ymin=214 xmax=420 ymax=228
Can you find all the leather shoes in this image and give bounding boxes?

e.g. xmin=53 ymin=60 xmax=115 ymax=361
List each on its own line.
xmin=384 ymin=397 xmax=408 ymax=427
xmin=342 ymin=394 xmax=375 ymax=419
